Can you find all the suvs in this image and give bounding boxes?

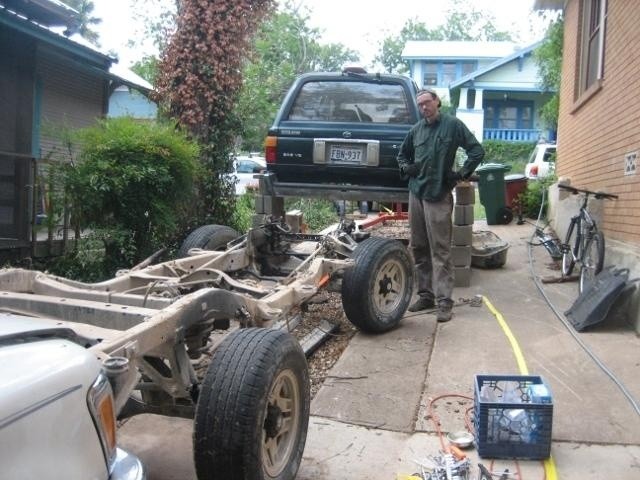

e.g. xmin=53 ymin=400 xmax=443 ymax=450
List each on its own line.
xmin=265 ymin=67 xmax=420 ymax=188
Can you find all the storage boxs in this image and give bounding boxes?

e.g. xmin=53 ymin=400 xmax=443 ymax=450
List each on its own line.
xmin=474 ymin=376 xmax=553 ymax=461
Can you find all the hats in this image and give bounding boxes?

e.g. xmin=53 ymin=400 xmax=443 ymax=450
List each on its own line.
xmin=415 ymin=88 xmax=441 ymax=108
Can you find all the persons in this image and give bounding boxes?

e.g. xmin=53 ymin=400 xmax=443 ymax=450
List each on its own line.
xmin=396 ymin=88 xmax=486 ymax=323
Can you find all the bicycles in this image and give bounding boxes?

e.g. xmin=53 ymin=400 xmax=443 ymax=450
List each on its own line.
xmin=558 ymin=183 xmax=619 ymax=294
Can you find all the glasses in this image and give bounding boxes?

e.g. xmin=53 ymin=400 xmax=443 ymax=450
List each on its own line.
xmin=417 ymin=98 xmax=435 ymax=107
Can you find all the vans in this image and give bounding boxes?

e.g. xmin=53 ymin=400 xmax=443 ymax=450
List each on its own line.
xmin=525 ymin=145 xmax=559 ymax=179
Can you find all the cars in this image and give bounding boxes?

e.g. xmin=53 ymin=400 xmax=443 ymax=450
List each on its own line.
xmin=218 ymin=159 xmax=265 ymax=195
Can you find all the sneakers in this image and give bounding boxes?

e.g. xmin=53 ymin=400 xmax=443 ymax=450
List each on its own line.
xmin=408 ymin=293 xmax=436 ymax=312
xmin=436 ymin=300 xmax=453 ymax=322
